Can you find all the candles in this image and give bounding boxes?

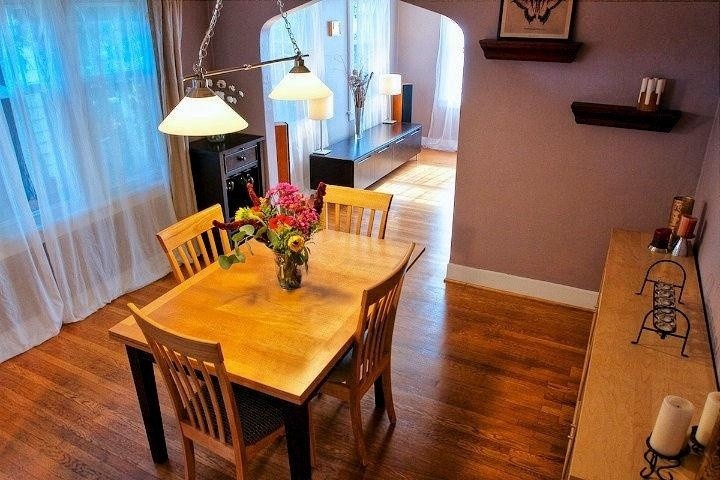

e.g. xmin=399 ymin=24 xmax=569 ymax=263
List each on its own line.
xmin=649 ymin=395 xmax=695 ymax=456
xmin=652 ymin=227 xmax=671 ymax=248
xmin=696 ymin=391 xmax=720 ymax=447
xmin=678 ymin=213 xmax=699 ymax=239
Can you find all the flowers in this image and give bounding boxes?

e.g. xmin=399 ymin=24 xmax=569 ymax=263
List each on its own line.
xmin=214 ymin=181 xmax=327 ymax=271
xmin=342 ymin=57 xmax=374 ymax=139
xmin=207 ymin=73 xmax=246 ymax=108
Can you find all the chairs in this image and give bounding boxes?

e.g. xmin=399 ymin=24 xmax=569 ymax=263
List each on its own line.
xmin=127 ymin=302 xmax=318 ymax=479
xmin=155 ymin=204 xmax=234 ymax=284
xmin=318 ymin=242 xmax=418 ymax=475
xmin=312 ymin=182 xmax=394 ymax=244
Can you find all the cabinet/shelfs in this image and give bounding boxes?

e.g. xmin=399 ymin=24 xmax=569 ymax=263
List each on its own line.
xmin=185 ymin=132 xmax=268 ymax=259
xmin=309 ymin=120 xmax=422 ymax=191
xmin=477 ymin=34 xmax=683 ymax=136
xmin=561 ymin=224 xmax=720 ymax=480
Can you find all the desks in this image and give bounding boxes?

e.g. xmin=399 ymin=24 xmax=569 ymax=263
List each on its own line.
xmin=109 ymin=223 xmax=425 ymax=478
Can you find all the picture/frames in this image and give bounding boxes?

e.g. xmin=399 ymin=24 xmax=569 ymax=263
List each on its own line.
xmin=496 ymin=0 xmax=578 ymax=41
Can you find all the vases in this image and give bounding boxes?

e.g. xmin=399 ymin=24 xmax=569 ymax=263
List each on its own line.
xmin=204 ymin=130 xmax=227 ymax=142
xmin=350 ymin=105 xmax=365 ymax=138
xmin=273 ymin=251 xmax=305 ymax=291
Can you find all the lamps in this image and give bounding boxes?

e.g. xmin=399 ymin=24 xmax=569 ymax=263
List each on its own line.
xmin=306 ymin=91 xmax=337 ymax=155
xmin=379 ymin=72 xmax=402 ymax=125
xmin=157 ymin=0 xmax=334 ymax=137
